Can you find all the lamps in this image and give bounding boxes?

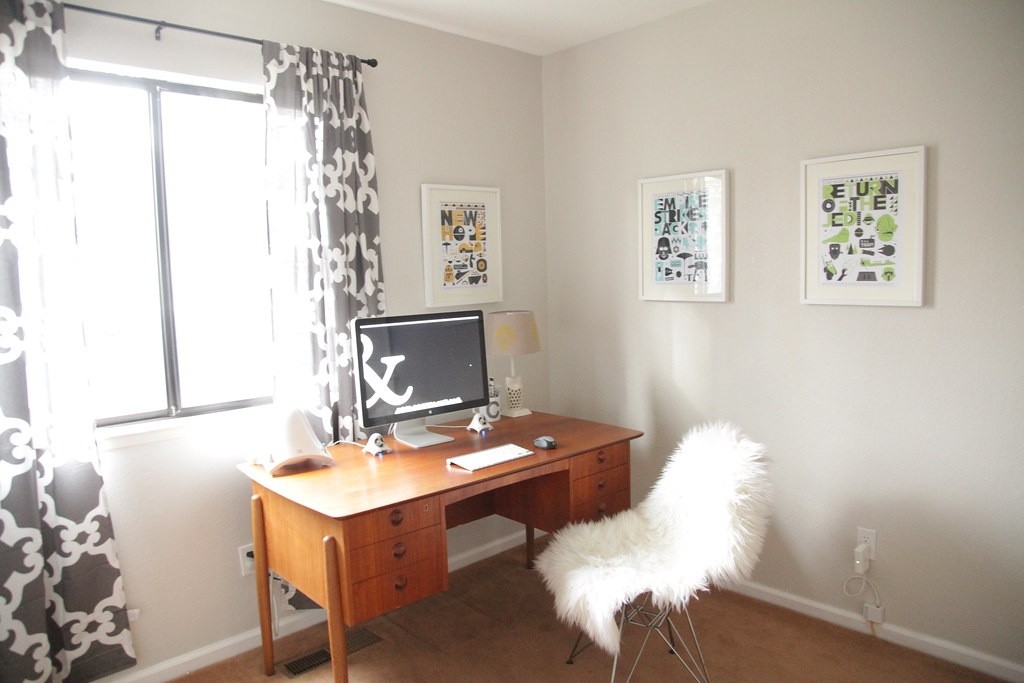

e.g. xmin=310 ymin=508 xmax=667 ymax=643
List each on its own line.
xmin=485 ymin=310 xmax=544 ymax=418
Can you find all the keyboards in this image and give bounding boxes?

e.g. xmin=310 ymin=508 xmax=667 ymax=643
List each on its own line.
xmin=447 ymin=443 xmax=535 ymax=472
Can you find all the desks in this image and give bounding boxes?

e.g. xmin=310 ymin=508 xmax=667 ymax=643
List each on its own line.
xmin=235 ymin=410 xmax=644 ymax=683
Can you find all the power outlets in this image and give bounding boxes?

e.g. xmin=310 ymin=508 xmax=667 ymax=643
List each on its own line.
xmin=239 ymin=543 xmax=256 ymax=576
xmin=856 ymin=526 xmax=876 ymax=561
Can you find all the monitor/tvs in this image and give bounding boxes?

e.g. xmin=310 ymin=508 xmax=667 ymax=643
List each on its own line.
xmin=351 ymin=309 xmax=489 ymax=447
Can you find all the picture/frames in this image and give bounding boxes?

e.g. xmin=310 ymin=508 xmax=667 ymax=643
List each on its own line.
xmin=420 ymin=184 xmax=504 ymax=308
xmin=638 ymin=169 xmax=731 ymax=303
xmin=799 ymin=145 xmax=928 ymax=307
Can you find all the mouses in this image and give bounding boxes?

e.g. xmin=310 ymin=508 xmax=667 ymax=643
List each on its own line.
xmin=533 ymin=435 xmax=556 ymax=449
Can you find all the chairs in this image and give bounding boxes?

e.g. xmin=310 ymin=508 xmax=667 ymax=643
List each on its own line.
xmin=530 ymin=419 xmax=773 ymax=683
